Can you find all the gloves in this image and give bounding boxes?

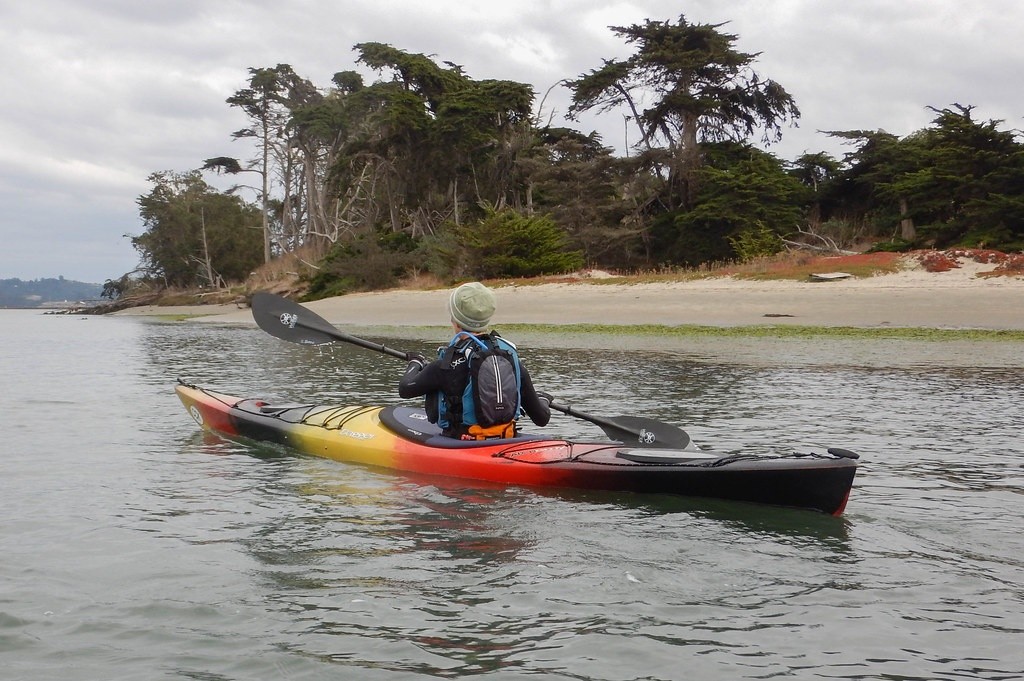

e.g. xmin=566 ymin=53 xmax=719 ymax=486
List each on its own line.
xmin=535 ymin=390 xmax=556 ymax=407
xmin=406 ymin=351 xmax=427 ymax=372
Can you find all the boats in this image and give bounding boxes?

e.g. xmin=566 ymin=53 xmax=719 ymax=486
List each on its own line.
xmin=175 ymin=380 xmax=856 ymax=518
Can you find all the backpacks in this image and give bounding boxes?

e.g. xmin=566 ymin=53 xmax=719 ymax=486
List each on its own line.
xmin=470 ymin=337 xmax=519 ymax=429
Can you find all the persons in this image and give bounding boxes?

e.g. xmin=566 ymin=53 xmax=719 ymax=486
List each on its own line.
xmin=399 ymin=281 xmax=553 ymax=441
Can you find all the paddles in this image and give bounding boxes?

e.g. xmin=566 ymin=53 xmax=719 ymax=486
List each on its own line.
xmin=249 ymin=289 xmax=689 ymax=446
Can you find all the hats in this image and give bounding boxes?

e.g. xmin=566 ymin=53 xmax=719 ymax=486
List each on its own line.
xmin=448 ymin=282 xmax=496 ymax=332
